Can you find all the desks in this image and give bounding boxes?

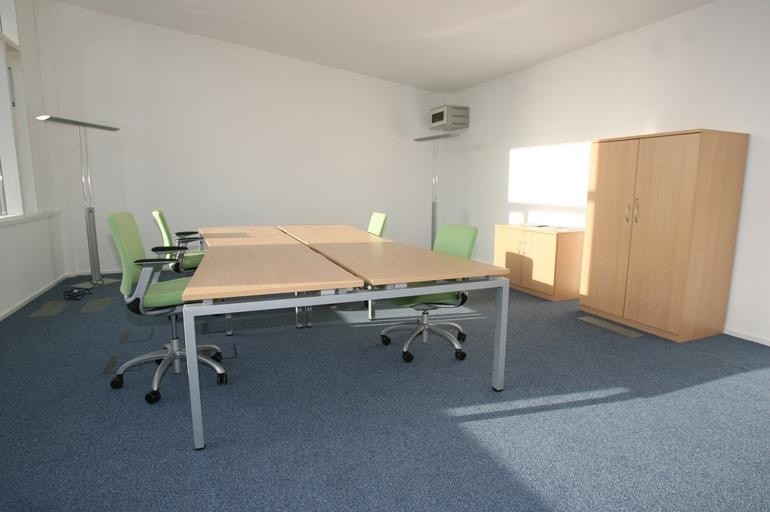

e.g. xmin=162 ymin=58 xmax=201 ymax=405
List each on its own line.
xmin=494 ymin=224 xmax=582 ymax=302
xmin=181 ymin=224 xmax=509 ymax=451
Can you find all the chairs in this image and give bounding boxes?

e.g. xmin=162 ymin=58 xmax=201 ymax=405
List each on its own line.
xmin=368 ymin=212 xmax=387 ymax=237
xmin=379 ymin=223 xmax=477 ymax=362
xmin=152 ymin=209 xmax=205 ymax=270
xmin=106 ymin=211 xmax=227 ymax=403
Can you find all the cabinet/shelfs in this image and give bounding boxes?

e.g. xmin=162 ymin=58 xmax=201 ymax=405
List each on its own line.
xmin=578 ymin=129 xmax=750 ymax=343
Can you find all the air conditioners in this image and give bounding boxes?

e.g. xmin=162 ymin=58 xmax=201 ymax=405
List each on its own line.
xmin=428 ymin=105 xmax=470 ymax=131
